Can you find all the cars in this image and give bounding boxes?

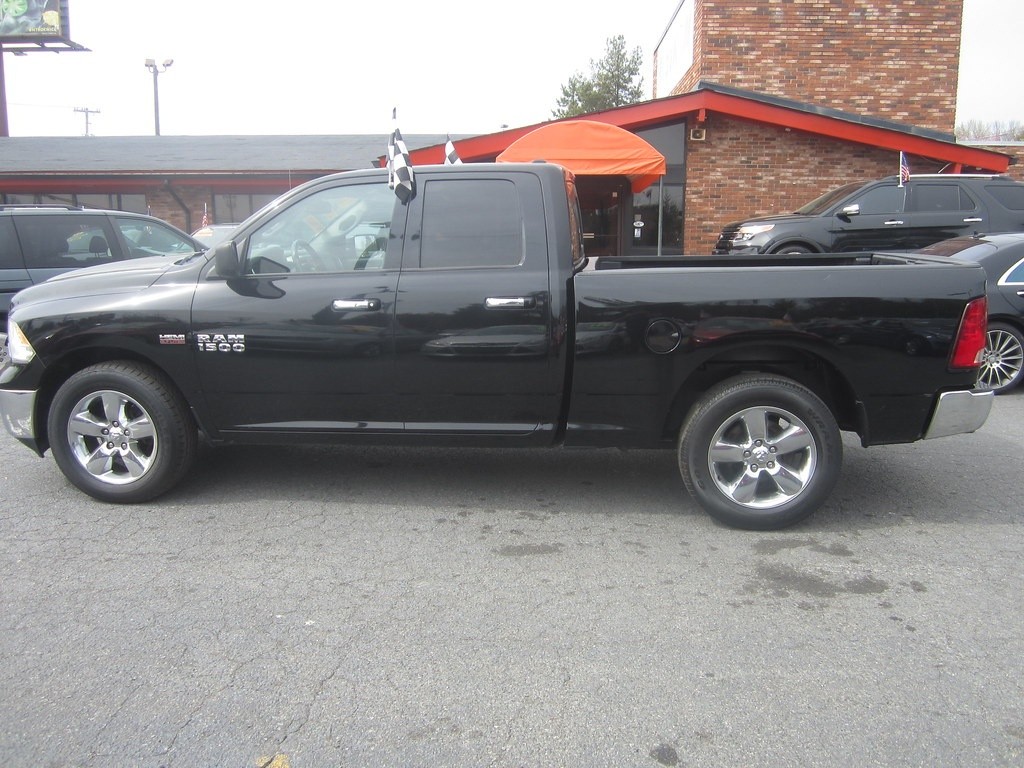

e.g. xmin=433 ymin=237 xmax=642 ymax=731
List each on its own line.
xmin=908 ymin=232 xmax=1024 ymax=396
xmin=174 ymin=222 xmax=244 ymax=254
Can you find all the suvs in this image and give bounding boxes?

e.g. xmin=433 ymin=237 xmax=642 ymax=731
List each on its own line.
xmin=711 ymin=168 xmax=1022 ymax=256
xmin=0 ymin=200 xmax=208 ymax=329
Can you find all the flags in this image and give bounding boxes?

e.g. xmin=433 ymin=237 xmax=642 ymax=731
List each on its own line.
xmin=202 ymin=203 xmax=209 ymax=227
xmin=444 ymin=134 xmax=463 ymax=164
xmin=387 ymin=107 xmax=414 ymax=205
xmin=900 ymin=150 xmax=910 ymax=184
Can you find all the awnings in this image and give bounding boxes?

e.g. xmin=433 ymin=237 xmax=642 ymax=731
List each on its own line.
xmin=495 ymin=120 xmax=666 ymax=257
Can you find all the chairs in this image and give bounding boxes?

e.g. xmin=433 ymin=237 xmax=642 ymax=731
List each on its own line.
xmin=47 ymin=237 xmax=79 ymax=267
xmin=84 ymin=235 xmax=112 ymax=263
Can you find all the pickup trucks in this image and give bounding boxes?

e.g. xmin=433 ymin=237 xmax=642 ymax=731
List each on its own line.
xmin=0 ymin=159 xmax=994 ymax=528
